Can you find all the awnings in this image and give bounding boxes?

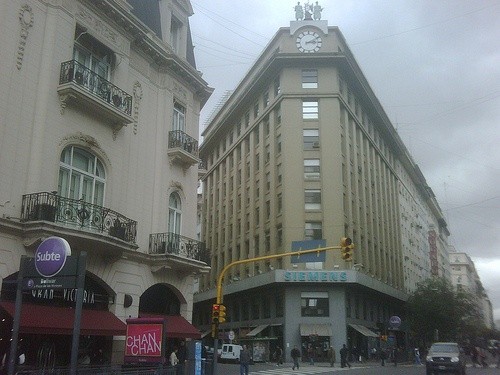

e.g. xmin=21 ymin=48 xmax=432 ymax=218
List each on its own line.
xmin=347 ymin=324 xmax=378 ymax=337
xmin=246 ymin=325 xmax=268 ymax=336
xmin=137 ymin=313 xmax=201 ymax=339
xmin=0 ymin=301 xmax=130 ymax=336
xmin=300 ymin=324 xmax=332 ymax=337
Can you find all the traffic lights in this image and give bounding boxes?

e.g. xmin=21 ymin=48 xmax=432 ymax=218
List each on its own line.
xmin=220 ymin=305 xmax=226 ymax=323
xmin=211 ymin=304 xmax=219 ymax=324
xmin=341 ymin=237 xmax=352 ymax=260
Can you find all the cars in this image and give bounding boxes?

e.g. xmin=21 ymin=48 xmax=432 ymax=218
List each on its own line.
xmin=425 ymin=342 xmax=467 ymax=375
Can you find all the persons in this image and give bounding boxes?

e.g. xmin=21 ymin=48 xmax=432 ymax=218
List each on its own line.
xmin=327 ymin=344 xmax=336 ymax=367
xmin=313 ymin=1 xmax=324 ymax=20
xmin=340 ymin=344 xmax=348 ymax=368
xmin=391 ymin=345 xmax=399 ymax=367
xmin=414 ymin=346 xmax=424 ymax=368
xmin=371 ymin=346 xmax=377 ymax=361
xmin=169 ymin=347 xmax=179 ymax=375
xmin=295 ymin=1 xmax=303 ymax=20
xmin=481 ymin=347 xmax=490 ymax=368
xmin=240 ymin=344 xmax=251 ymax=375
xmin=273 ymin=345 xmax=283 ymax=367
xmin=290 ymin=344 xmax=301 ymax=370
xmin=470 ymin=346 xmax=481 ymax=367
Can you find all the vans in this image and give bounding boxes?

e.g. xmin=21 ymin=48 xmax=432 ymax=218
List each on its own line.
xmin=219 ymin=344 xmax=243 ymax=363
